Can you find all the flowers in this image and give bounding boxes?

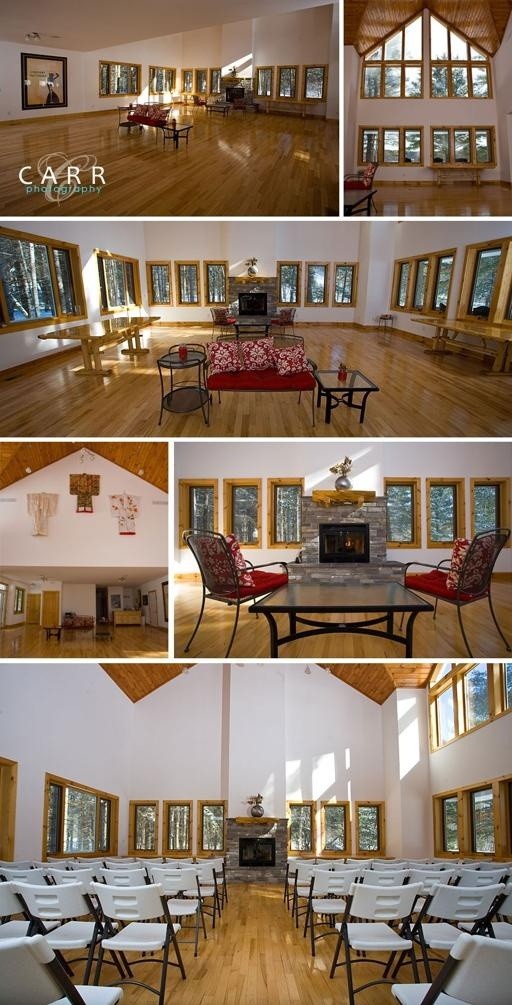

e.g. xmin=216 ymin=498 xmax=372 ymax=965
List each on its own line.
xmin=248 ymin=793 xmax=263 ymax=806
xmin=229 ymin=66 xmax=237 ymax=72
xmin=330 ymin=455 xmax=352 ymax=475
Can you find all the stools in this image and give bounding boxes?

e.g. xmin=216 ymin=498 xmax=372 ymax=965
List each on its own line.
xmin=376 ymin=315 xmax=393 ymax=331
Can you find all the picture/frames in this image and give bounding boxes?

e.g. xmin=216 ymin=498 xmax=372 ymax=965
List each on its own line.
xmin=162 ymin=581 xmax=168 ymax=622
xmin=111 ymin=595 xmax=121 ymax=608
xmin=20 ymin=53 xmax=68 ymax=109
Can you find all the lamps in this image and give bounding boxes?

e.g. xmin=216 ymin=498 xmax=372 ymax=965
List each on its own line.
xmin=117 ymin=576 xmax=126 ymax=584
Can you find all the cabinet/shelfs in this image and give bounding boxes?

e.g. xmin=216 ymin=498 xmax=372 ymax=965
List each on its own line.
xmin=157 ymin=343 xmax=210 ymax=426
xmin=113 ymin=610 xmax=142 ymax=628
xmin=116 ymin=105 xmax=139 ymax=134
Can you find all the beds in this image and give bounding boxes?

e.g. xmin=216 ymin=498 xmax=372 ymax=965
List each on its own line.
xmin=162 ymin=123 xmax=193 ymax=149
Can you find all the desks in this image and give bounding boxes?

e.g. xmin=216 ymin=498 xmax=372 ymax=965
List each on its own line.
xmin=313 ymin=370 xmax=379 ymax=424
xmin=428 ymin=165 xmax=485 ymax=185
xmin=249 ymin=583 xmax=434 ymax=657
xmin=37 ymin=317 xmax=162 ymax=375
xmin=43 ymin=624 xmax=62 ymax=640
xmin=259 ymin=98 xmax=317 ymax=117
xmin=411 ymin=319 xmax=512 ymax=376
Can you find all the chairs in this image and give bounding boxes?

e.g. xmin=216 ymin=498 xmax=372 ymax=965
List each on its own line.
xmin=399 ymin=527 xmax=512 ymax=657
xmin=282 ymin=858 xmax=512 ymax=1005
xmin=192 ymin=95 xmax=247 ymax=119
xmin=344 ymin=161 xmax=379 ymax=214
xmin=210 ymin=307 xmax=296 ymax=337
xmin=182 ymin=529 xmax=288 ymax=658
xmin=0 ymin=855 xmax=228 ymax=1005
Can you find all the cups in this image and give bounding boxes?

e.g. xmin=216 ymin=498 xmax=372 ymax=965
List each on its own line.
xmin=74 ymin=304 xmax=81 ymax=315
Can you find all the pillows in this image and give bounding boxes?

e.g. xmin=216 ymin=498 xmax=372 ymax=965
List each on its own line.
xmin=446 ymin=536 xmax=496 ymax=594
xmin=206 ymin=337 xmax=312 ymax=376
xmin=194 ymin=535 xmax=255 ymax=589
xmin=133 ymin=104 xmax=170 ymax=120
xmin=364 ymin=163 xmax=375 ymax=186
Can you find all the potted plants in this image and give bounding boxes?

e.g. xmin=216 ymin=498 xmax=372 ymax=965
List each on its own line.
xmin=337 ymin=363 xmax=348 ymax=381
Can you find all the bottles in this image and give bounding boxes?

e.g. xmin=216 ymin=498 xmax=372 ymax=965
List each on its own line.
xmin=129 ymin=103 xmax=132 ymax=110
xmin=178 ymin=343 xmax=187 ymax=359
xmin=172 ymin=118 xmax=177 ymax=129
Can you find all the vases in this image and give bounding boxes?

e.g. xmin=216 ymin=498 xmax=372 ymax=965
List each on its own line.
xmin=251 ymin=806 xmax=264 ymax=817
xmin=231 ymin=72 xmax=236 ymax=77
xmin=335 ymin=475 xmax=350 ymax=489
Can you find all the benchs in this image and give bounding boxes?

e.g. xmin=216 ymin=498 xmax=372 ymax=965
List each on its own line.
xmin=202 ymin=331 xmax=318 ymax=428
xmin=127 ymin=102 xmax=172 ymax=144
xmin=59 ymin=615 xmax=94 ymax=639
